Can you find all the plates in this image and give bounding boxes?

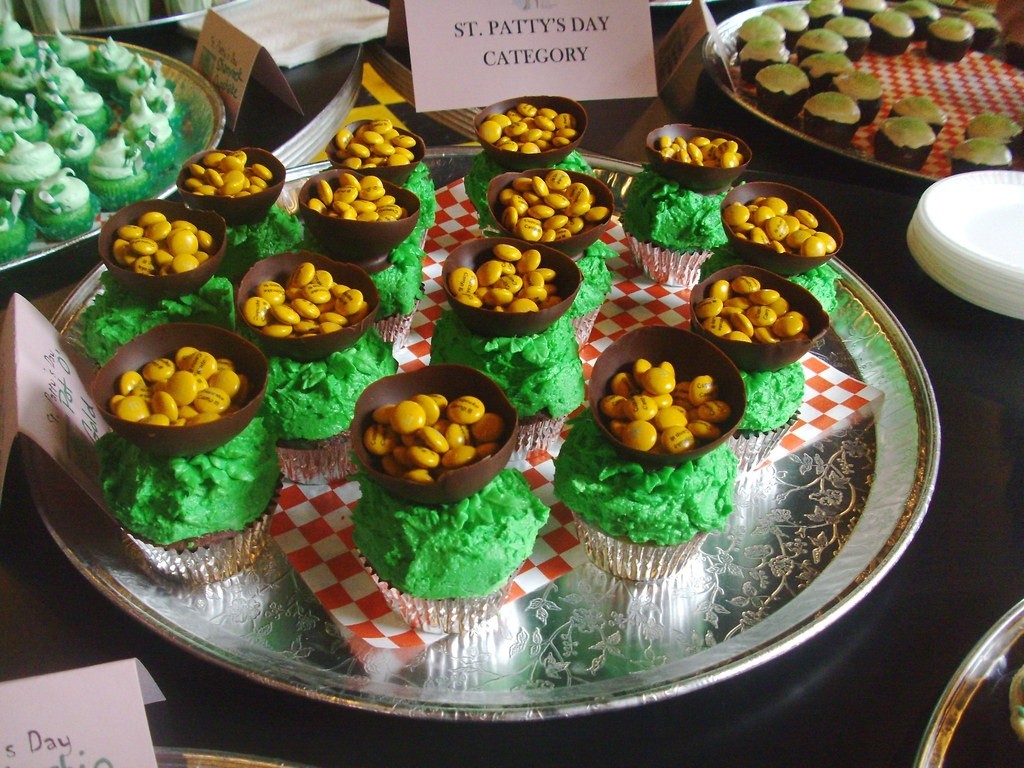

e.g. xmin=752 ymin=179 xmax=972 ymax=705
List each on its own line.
xmin=30 ymin=138 xmax=940 ymax=719
xmin=905 ymin=170 xmax=1024 ymax=322
xmin=4 ymin=36 xmax=227 ymax=273
xmin=712 ymin=2 xmax=1024 ymax=171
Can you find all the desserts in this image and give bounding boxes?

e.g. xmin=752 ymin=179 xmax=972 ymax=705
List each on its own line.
xmin=0 ymin=1 xmax=1024 ymax=636
